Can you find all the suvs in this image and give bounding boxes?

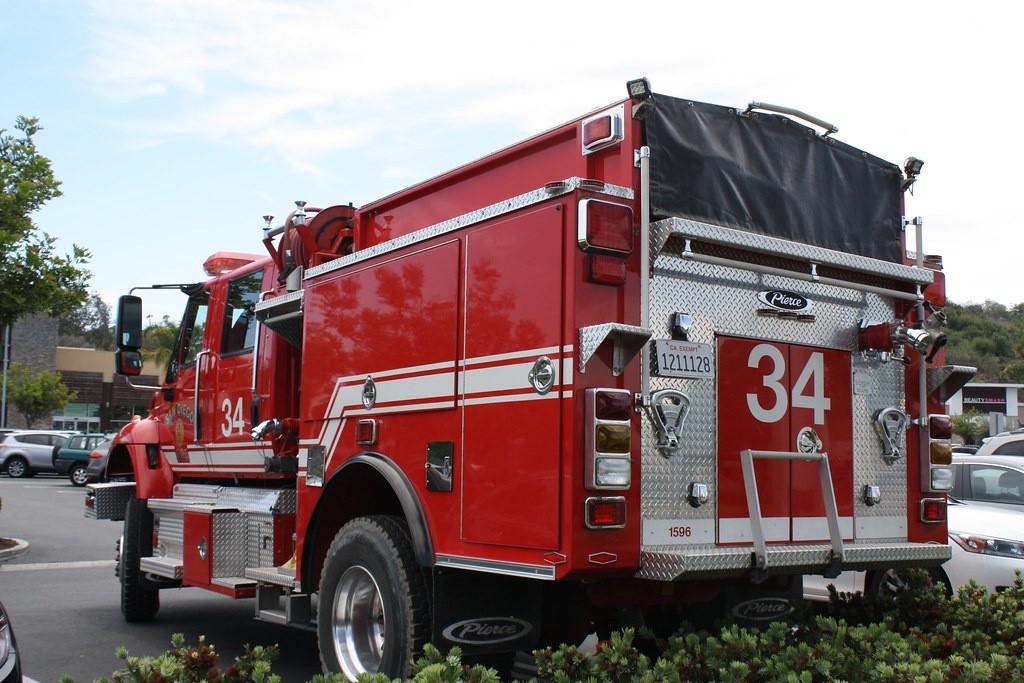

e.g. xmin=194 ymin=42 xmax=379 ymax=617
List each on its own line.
xmin=0 ymin=431 xmax=117 ymax=487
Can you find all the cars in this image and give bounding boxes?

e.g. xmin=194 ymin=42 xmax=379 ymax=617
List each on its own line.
xmin=804 ymin=427 xmax=1024 ymax=618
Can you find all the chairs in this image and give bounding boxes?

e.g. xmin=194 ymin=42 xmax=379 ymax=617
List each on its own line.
xmin=999 ymin=473 xmax=1018 ymax=500
xmin=974 ymin=476 xmax=987 ymax=497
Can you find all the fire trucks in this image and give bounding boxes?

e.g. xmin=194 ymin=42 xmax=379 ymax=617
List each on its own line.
xmin=84 ymin=77 xmax=978 ymax=682
